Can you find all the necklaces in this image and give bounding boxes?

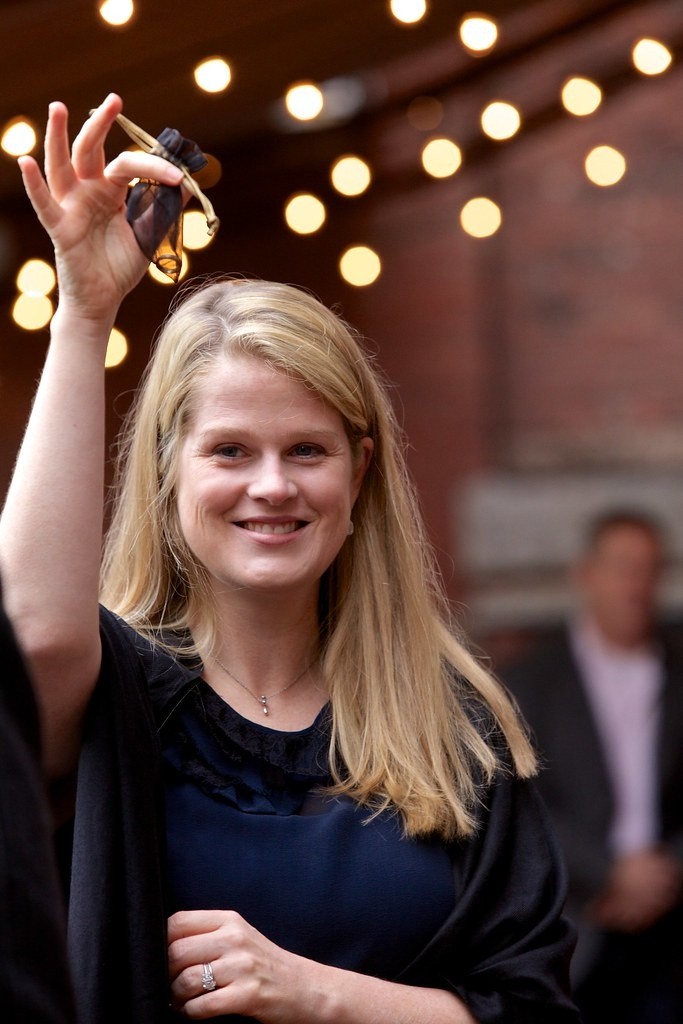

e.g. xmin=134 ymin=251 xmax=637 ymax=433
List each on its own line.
xmin=213 ymin=646 xmax=323 ymax=717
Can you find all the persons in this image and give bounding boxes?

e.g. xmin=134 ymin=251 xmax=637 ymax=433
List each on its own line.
xmin=0 ymin=92 xmax=578 ymax=1024
xmin=493 ymin=509 xmax=683 ymax=1024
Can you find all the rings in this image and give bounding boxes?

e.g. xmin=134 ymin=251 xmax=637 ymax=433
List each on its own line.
xmin=202 ymin=963 xmax=216 ymax=991
xmin=156 ymin=254 xmax=182 ymax=274
